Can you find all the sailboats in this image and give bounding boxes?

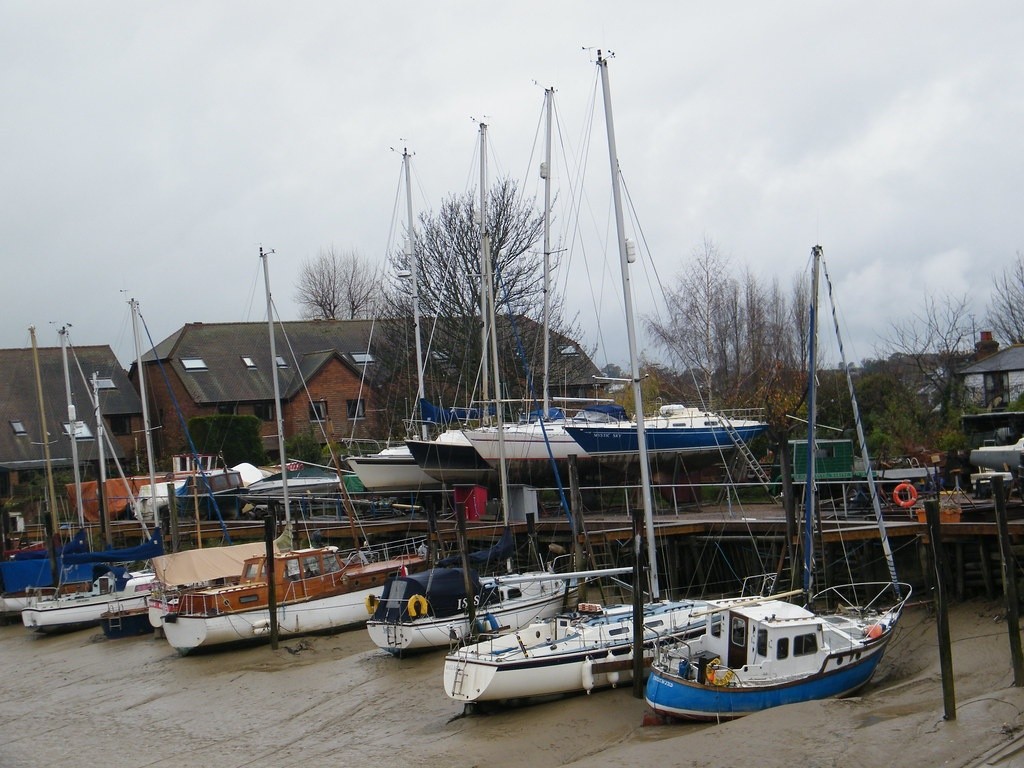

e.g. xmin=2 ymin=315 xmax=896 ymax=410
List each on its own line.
xmin=0 ymin=244 xmax=434 ymax=656
xmin=440 ymin=42 xmax=783 ymax=705
xmin=643 ymin=239 xmax=911 ymax=723
xmin=336 ymin=91 xmax=769 ymax=485
xmin=365 ymin=119 xmax=590 ymax=659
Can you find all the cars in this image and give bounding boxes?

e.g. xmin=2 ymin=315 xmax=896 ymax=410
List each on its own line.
xmin=968 ymin=439 xmax=1023 ymax=481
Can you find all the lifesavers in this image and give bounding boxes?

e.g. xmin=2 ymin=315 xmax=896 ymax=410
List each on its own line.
xmin=408 ymin=594 xmax=428 ymax=616
xmin=893 ymin=483 xmax=918 ymax=508
xmin=365 ymin=594 xmax=379 ymax=614
xmin=297 ymin=464 xmax=304 ymax=471
xmin=706 ymin=657 xmax=734 ymax=687
